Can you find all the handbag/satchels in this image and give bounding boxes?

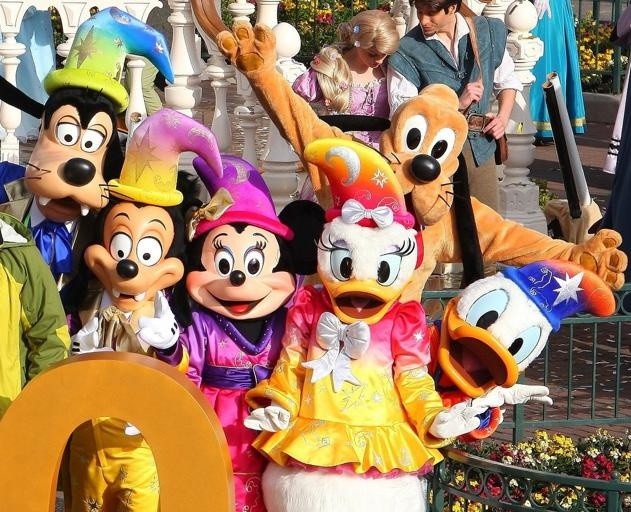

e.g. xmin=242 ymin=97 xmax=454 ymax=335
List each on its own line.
xmin=497 ymin=132 xmax=509 ymax=165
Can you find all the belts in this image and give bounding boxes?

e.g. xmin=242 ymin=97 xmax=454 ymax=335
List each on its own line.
xmin=463 ymin=113 xmax=493 ymax=134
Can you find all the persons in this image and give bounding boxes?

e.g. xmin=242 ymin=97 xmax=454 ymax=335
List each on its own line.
xmin=1 ymin=209 xmax=71 ymax=416
xmin=386 ymin=2 xmax=525 ymax=213
xmin=13 ymin=6 xmax=58 ymax=145
xmin=527 ymin=0 xmax=587 ymax=146
xmin=292 ymin=8 xmax=402 ymax=154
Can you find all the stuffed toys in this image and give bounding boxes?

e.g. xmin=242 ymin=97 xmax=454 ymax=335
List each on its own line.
xmin=138 ymin=152 xmax=297 ymax=512
xmin=425 ymin=255 xmax=618 ymax=441
xmin=239 ymin=135 xmax=482 ymax=512
xmin=1 ymin=5 xmax=176 ymax=335
xmin=217 ymin=18 xmax=630 ymax=292
xmin=59 ymin=105 xmax=225 ymax=511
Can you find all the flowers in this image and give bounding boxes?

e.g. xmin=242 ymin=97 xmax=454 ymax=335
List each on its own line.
xmin=277 ymin=1 xmax=396 ymax=56
xmin=574 ymin=11 xmax=628 ymax=94
xmin=428 ymin=429 xmax=630 ymax=511
